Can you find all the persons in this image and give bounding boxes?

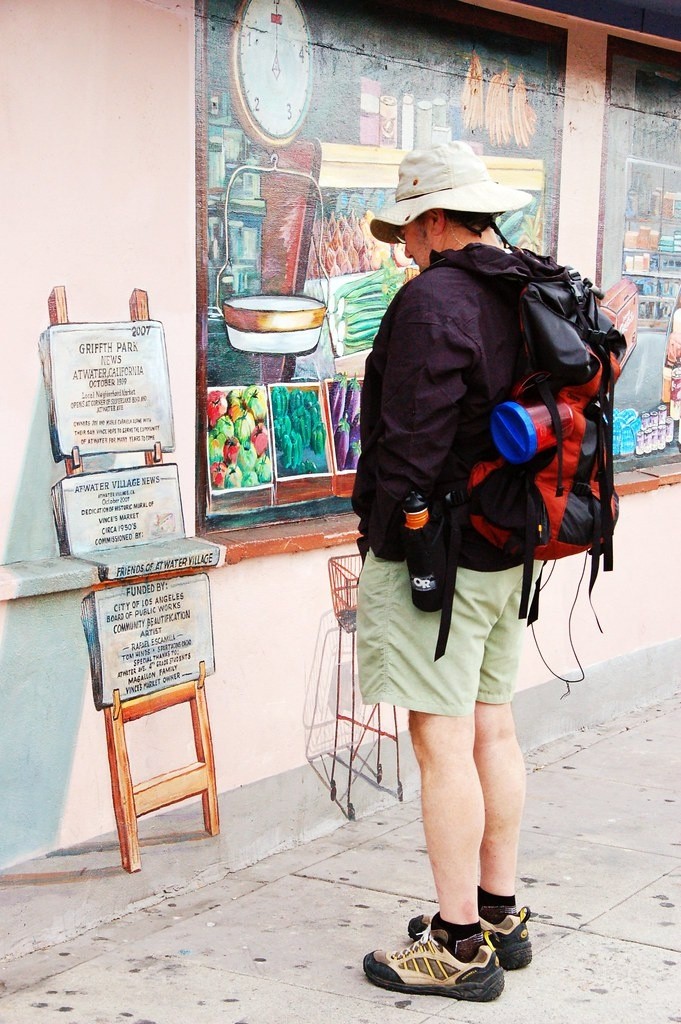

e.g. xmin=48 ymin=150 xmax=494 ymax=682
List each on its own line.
xmin=350 ymin=141 xmax=545 ymax=1003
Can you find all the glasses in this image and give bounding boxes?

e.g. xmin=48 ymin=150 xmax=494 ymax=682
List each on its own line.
xmin=393 ymin=224 xmax=406 ymax=244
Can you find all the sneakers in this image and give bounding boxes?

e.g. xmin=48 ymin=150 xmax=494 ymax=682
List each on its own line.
xmin=363 ymin=923 xmax=504 ymax=1003
xmin=407 ymin=897 xmax=531 ymax=973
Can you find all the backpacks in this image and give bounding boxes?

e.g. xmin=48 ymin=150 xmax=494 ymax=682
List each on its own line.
xmin=421 ymin=257 xmax=627 ymax=560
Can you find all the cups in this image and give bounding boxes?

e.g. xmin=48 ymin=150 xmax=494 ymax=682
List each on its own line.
xmin=490 ymin=397 xmax=574 ymax=464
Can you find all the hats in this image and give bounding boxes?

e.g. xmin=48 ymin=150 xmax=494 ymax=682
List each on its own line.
xmin=370 ymin=141 xmax=533 ymax=243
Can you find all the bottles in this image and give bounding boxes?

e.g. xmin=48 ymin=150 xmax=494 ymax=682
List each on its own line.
xmin=403 ymin=491 xmax=430 ymax=528
